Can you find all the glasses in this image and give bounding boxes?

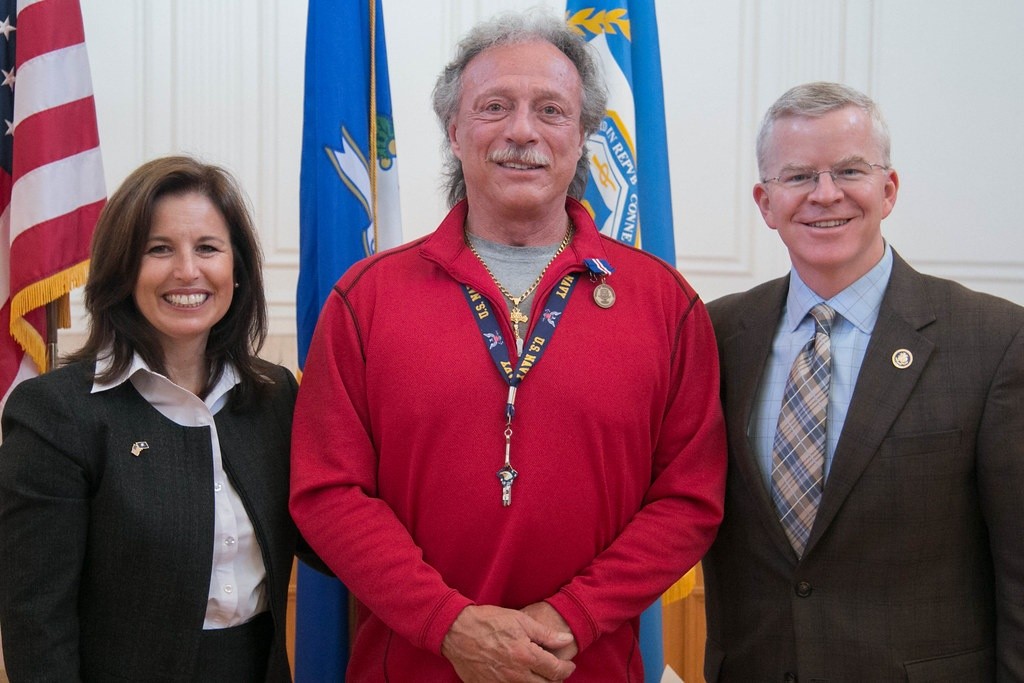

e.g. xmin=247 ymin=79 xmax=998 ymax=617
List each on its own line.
xmin=761 ymin=163 xmax=887 ymax=189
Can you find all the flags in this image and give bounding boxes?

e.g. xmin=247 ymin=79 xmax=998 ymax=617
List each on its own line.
xmin=297 ymin=0 xmax=401 ymax=683
xmin=0 ymin=0 xmax=106 ymax=405
xmin=565 ymin=0 xmax=707 ymax=683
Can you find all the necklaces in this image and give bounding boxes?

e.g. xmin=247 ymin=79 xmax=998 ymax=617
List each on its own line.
xmin=465 ymin=224 xmax=572 ymax=358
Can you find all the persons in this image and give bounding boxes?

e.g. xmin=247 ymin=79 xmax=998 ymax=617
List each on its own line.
xmin=0 ymin=156 xmax=335 ymax=683
xmin=290 ymin=16 xmax=726 ymax=682
xmin=702 ymin=83 xmax=1024 ymax=683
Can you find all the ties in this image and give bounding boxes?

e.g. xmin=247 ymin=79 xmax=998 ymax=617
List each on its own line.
xmin=771 ymin=302 xmax=837 ymax=559
